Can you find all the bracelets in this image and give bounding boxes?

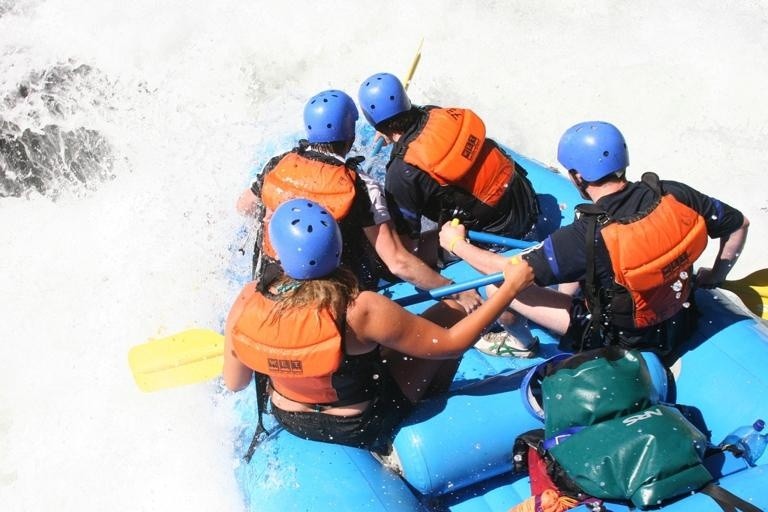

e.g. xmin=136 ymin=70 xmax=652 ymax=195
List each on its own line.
xmin=450 ymin=236 xmax=464 ymax=252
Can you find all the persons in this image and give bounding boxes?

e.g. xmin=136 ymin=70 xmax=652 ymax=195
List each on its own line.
xmin=436 ymin=120 xmax=751 ymax=361
xmin=219 ymin=198 xmax=536 ymax=452
xmin=358 ymin=71 xmax=543 ymax=272
xmin=234 ymin=88 xmax=487 ymax=318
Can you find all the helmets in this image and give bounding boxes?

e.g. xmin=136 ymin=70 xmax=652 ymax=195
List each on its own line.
xmin=303 ymin=89 xmax=359 ymax=145
xmin=268 ymin=198 xmax=343 ymax=281
xmin=557 ymin=120 xmax=630 ymax=183
xmin=358 ymin=72 xmax=411 ymax=128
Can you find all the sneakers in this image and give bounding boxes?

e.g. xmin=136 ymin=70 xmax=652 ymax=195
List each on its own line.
xmin=473 ymin=331 xmax=539 ymax=358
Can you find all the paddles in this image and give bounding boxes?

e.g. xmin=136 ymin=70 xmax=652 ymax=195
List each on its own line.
xmin=364 ymin=36 xmax=423 ymax=172
xmin=129 ymin=254 xmax=521 ymax=394
xmin=448 ymin=218 xmax=767 ymax=318
